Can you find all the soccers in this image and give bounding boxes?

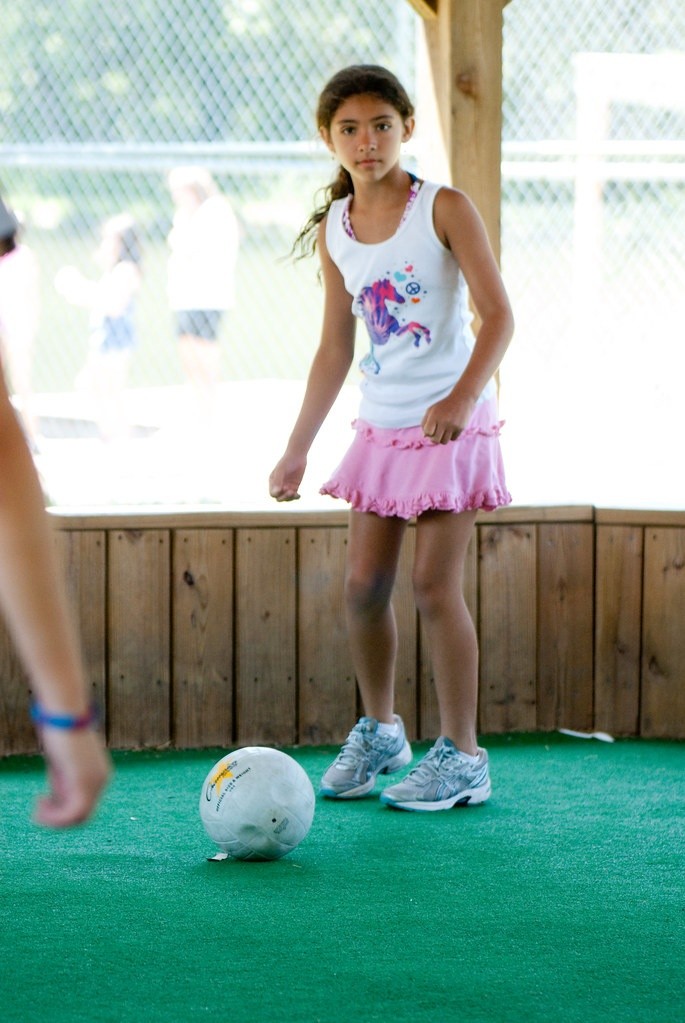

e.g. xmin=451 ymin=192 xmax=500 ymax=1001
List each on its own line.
xmin=200 ymin=742 xmax=316 ymax=865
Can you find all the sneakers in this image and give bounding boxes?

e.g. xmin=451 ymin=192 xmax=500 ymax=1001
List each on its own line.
xmin=380 ymin=736 xmax=491 ymax=812
xmin=318 ymin=715 xmax=413 ymax=799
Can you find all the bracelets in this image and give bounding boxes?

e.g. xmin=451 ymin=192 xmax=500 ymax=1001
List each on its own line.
xmin=30 ymin=695 xmax=107 ymax=732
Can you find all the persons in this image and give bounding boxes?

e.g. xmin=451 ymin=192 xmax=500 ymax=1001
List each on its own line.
xmin=60 ymin=212 xmax=148 ymax=398
xmin=1 ymin=166 xmax=117 ymax=833
xmin=267 ymin=64 xmax=521 ymax=818
xmin=164 ymin=162 xmax=241 ymax=379
